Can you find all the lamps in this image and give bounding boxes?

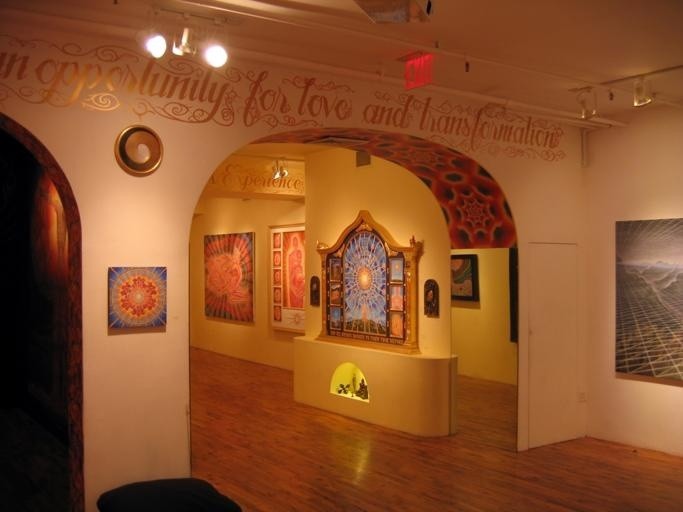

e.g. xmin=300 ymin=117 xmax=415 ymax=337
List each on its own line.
xmin=568 ymin=65 xmax=683 ymax=121
xmin=135 ymin=5 xmax=231 ymax=67
xmin=310 ymin=275 xmax=320 ymax=307
xmin=271 ymin=157 xmax=289 ymax=179
xmin=424 ymin=279 xmax=440 ymax=318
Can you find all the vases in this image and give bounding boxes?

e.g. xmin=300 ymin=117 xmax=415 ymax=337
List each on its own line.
xmin=265 ymin=223 xmax=304 ymax=334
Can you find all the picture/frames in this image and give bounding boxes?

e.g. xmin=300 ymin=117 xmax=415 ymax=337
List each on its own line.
xmin=451 ymin=254 xmax=480 ymax=301
